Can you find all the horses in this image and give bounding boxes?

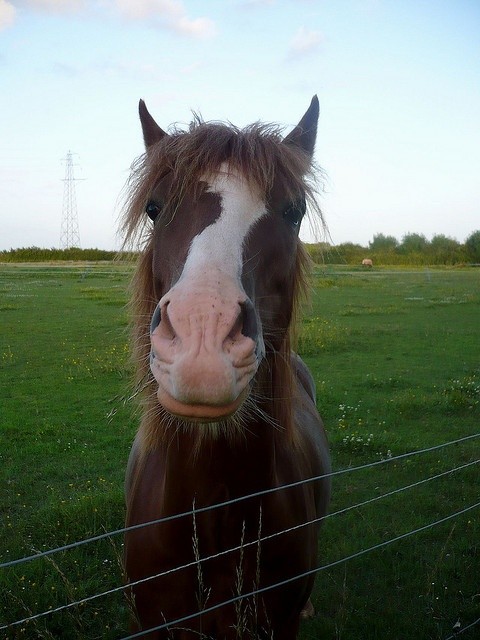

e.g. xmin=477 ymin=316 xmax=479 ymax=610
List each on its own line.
xmin=111 ymin=93 xmax=332 ymax=640
